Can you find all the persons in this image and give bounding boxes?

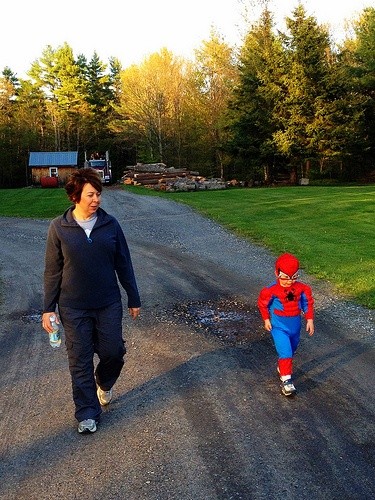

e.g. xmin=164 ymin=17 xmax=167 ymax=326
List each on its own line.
xmin=41 ymin=169 xmax=141 ymax=434
xmin=258 ymin=254 xmax=314 ymax=397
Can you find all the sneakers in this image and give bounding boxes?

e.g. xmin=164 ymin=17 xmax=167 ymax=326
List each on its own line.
xmin=280 ymin=375 xmax=296 ymax=396
xmin=276 ymin=366 xmax=280 ymax=373
xmin=77 ymin=418 xmax=97 ymax=433
xmin=96 ymin=381 xmax=113 ymax=405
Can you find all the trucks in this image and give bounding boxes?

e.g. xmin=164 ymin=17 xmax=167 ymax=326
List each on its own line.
xmin=83 ymin=151 xmax=113 ymax=186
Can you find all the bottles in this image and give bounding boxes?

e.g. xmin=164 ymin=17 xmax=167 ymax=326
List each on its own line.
xmin=49 ymin=316 xmax=61 ymax=348
